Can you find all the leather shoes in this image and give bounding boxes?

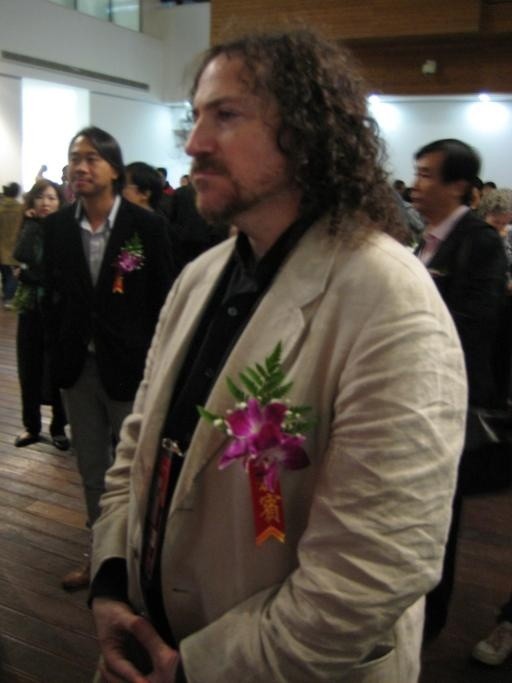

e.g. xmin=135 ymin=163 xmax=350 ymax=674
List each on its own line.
xmin=50 ymin=425 xmax=70 ymax=451
xmin=14 ymin=428 xmax=41 ymax=447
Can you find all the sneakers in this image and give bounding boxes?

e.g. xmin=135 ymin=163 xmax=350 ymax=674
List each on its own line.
xmin=61 ymin=560 xmax=91 ymax=591
xmin=474 ymin=622 xmax=510 ymax=662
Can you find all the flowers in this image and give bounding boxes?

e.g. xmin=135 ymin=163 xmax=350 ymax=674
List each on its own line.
xmin=192 ymin=339 xmax=317 ymax=495
xmin=115 ymin=230 xmax=150 ymax=273
